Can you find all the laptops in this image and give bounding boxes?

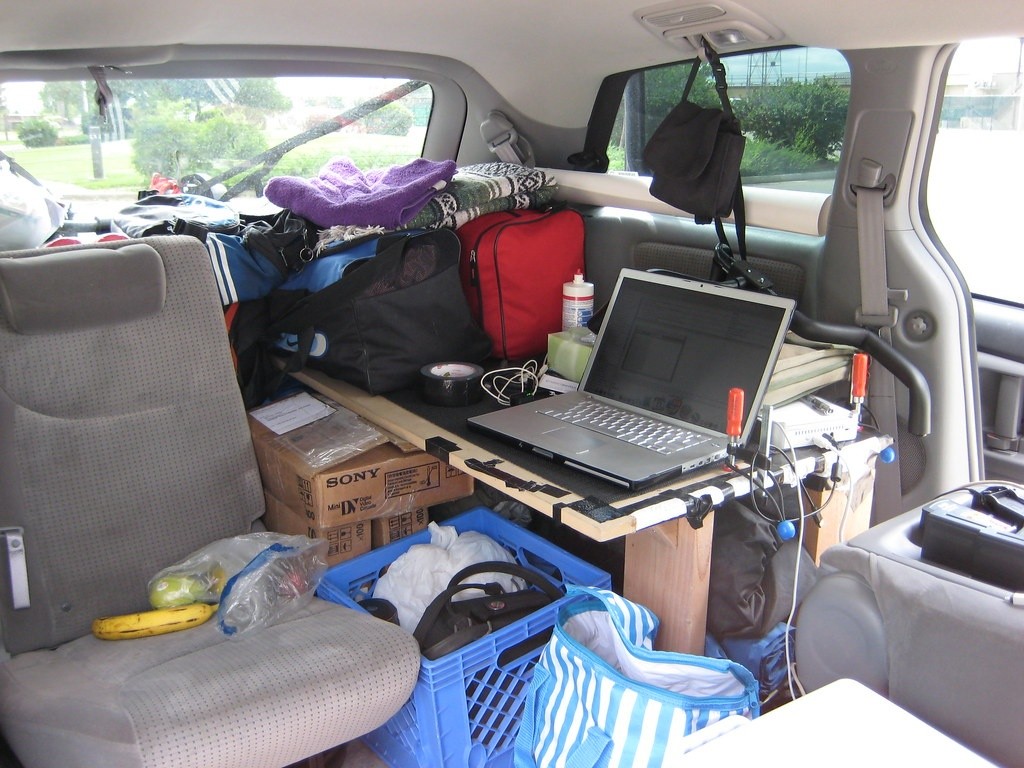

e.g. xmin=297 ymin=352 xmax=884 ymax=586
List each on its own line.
xmin=464 ymin=268 xmax=796 ymax=492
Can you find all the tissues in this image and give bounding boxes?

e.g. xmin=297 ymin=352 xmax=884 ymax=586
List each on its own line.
xmin=548 ymin=326 xmax=599 ymax=382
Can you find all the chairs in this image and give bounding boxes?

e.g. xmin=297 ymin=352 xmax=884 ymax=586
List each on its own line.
xmin=0 ymin=236 xmax=421 ymax=768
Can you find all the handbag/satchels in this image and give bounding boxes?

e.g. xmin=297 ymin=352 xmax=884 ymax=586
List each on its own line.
xmin=641 ymin=38 xmax=746 ymax=223
xmin=412 ymin=560 xmax=566 ymax=746
xmin=513 ymin=582 xmax=760 ymax=768
xmin=454 ymin=198 xmax=586 ymax=363
xmin=238 ymin=226 xmax=491 ymax=394
xmin=142 ymin=210 xmax=324 ymax=284
xmin=373 ymin=521 xmax=529 ymax=638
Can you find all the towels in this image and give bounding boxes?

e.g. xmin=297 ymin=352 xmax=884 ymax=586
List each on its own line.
xmin=264 ymin=157 xmax=459 ymax=228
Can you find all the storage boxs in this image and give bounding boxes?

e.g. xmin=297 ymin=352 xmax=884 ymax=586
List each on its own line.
xmin=316 ymin=504 xmax=614 ymax=768
xmin=660 ymin=677 xmax=1002 ymax=768
xmin=246 ymin=394 xmax=475 ymax=605
xmin=791 ymin=466 xmax=879 ymax=566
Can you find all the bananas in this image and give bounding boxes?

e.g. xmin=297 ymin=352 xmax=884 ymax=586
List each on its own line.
xmin=92 ymin=603 xmax=218 ymax=640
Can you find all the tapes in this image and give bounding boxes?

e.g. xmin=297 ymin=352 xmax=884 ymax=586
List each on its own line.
xmin=417 ymin=360 xmax=486 ymax=407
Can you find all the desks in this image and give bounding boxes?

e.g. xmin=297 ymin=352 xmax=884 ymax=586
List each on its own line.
xmin=288 ymin=364 xmax=895 ymax=661
